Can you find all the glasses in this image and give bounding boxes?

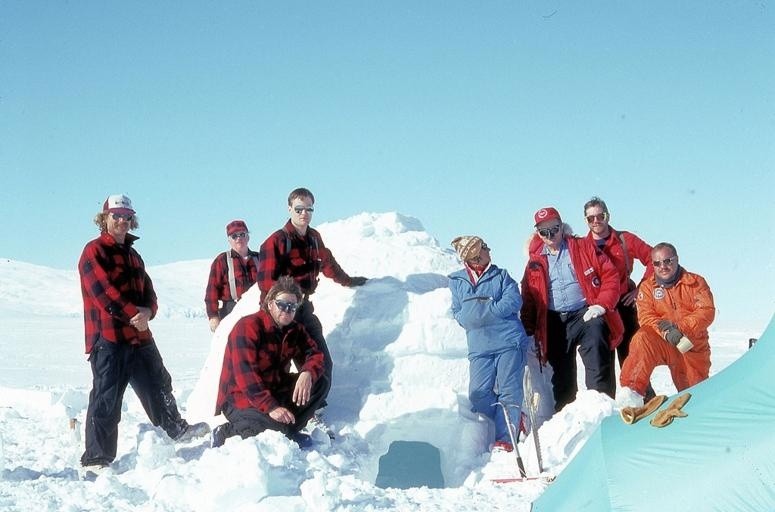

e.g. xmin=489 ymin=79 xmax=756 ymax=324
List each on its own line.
xmin=584 ymin=212 xmax=606 ymax=223
xmin=231 ymin=233 xmax=246 ymax=239
xmin=110 ymin=212 xmax=133 ymax=222
xmin=294 ymin=204 xmax=315 ymax=214
xmin=652 ymin=256 xmax=676 ymax=267
xmin=538 ymin=225 xmax=561 ymax=236
xmin=272 ymin=298 xmax=298 ymax=312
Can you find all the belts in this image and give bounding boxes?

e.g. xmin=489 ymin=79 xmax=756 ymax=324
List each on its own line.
xmin=547 ymin=309 xmax=574 ymax=314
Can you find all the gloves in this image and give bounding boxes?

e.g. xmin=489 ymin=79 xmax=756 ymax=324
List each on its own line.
xmin=349 ymin=276 xmax=367 ymax=287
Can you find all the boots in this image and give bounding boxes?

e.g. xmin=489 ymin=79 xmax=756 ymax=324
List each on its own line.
xmin=175 ymin=422 xmax=210 ymax=443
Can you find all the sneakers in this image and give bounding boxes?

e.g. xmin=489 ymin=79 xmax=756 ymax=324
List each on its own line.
xmin=519 ymin=407 xmax=532 ymax=436
xmin=305 ymin=414 xmax=335 ymax=439
xmin=211 ymin=424 xmax=227 ymax=448
xmin=288 ymin=430 xmax=312 ymax=447
xmin=491 ymin=441 xmax=514 ymax=453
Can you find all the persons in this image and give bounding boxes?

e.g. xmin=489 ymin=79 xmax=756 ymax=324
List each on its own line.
xmin=256 ymin=187 xmax=369 ymax=417
xmin=619 ymin=242 xmax=717 ymax=404
xmin=583 ymin=194 xmax=658 ymax=403
xmin=446 ymin=234 xmax=532 ymax=452
xmin=203 ymin=219 xmax=264 ymax=332
xmin=211 ymin=274 xmax=332 ymax=448
xmin=77 ymin=194 xmax=211 ymax=472
xmin=520 ymin=207 xmax=625 ymax=414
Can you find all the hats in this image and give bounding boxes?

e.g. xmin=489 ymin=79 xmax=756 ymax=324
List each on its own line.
xmin=103 ymin=193 xmax=138 ymax=215
xmin=534 ymin=207 xmax=560 ymax=227
xmin=451 ymin=235 xmax=483 ymax=261
xmin=225 ymin=220 xmax=248 ymax=234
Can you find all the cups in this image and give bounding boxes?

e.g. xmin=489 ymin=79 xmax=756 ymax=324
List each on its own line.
xmin=676 ymin=337 xmax=695 ymax=354
